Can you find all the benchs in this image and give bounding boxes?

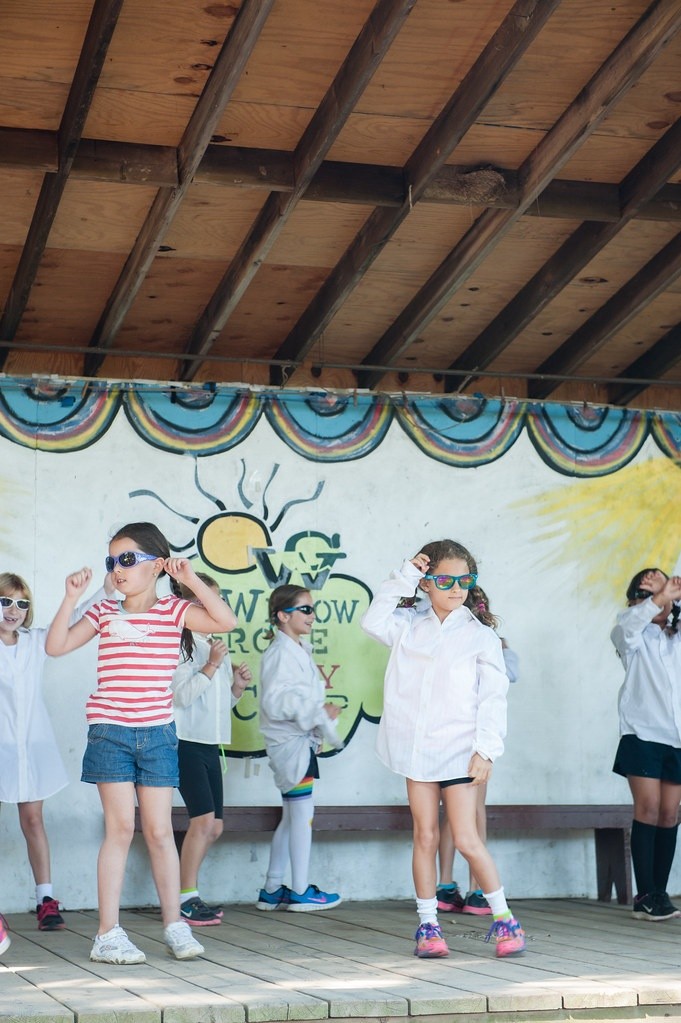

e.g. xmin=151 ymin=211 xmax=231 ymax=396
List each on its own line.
xmin=137 ymin=805 xmax=635 ymax=908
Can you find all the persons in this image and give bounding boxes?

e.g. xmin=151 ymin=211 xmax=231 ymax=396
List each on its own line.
xmin=0 ymin=570 xmax=117 ymax=931
xmin=174 ymin=571 xmax=251 ymax=927
xmin=43 ymin=521 xmax=242 ymax=962
xmin=362 ymin=539 xmax=525 ymax=955
xmin=613 ymin=567 xmax=681 ymax=921
xmin=257 ymin=586 xmax=340 ymax=912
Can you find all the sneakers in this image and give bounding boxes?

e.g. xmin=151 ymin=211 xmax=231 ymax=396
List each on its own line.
xmin=180 ymin=897 xmax=221 ymax=926
xmin=632 ymin=892 xmax=680 ymax=921
xmin=208 ymin=907 xmax=224 ymax=917
xmin=463 ymin=892 xmax=511 ymax=916
xmin=287 ymin=885 xmax=342 ymax=912
xmin=256 ymin=885 xmax=292 ymax=910
xmin=484 ymin=910 xmax=525 ymax=957
xmin=90 ymin=924 xmax=146 ymax=964
xmin=414 ymin=923 xmax=448 ymax=957
xmin=436 ymin=882 xmax=465 ymax=912
xmin=163 ymin=921 xmax=205 ymax=960
xmin=36 ymin=896 xmax=64 ymax=930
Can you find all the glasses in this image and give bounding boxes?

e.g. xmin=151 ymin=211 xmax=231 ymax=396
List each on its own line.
xmin=0 ymin=597 xmax=30 ymax=610
xmin=284 ymin=605 xmax=315 ymax=614
xmin=105 ymin=551 xmax=157 ymax=572
xmin=425 ymin=574 xmax=478 ymax=590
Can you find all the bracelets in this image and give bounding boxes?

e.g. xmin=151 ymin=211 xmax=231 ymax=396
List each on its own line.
xmin=198 ymin=671 xmax=210 ymax=680
xmin=207 ymin=661 xmax=219 ymax=668
